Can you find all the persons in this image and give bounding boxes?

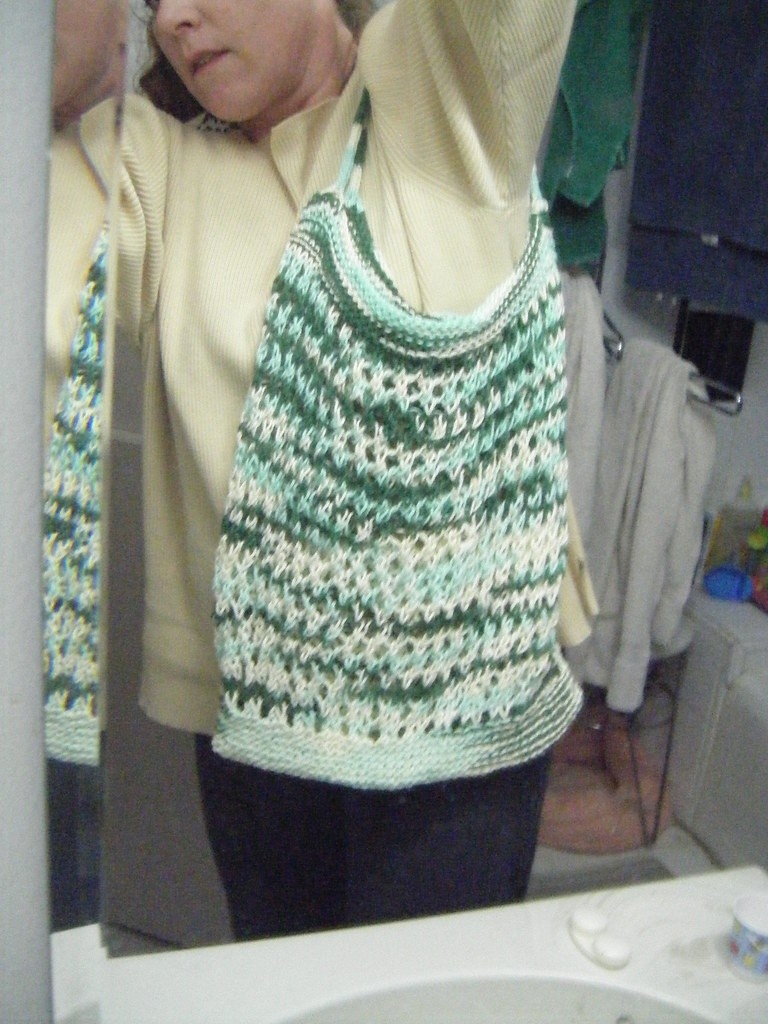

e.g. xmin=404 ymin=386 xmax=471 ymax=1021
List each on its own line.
xmin=40 ymin=1 xmax=115 ymax=931
xmin=119 ymin=1 xmax=601 ymax=943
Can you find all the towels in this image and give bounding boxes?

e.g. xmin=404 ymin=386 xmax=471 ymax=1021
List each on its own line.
xmin=621 ymin=0 xmax=768 ymax=327
xmin=534 ymin=2 xmax=652 ymax=323
xmin=553 ymin=271 xmax=727 ymax=719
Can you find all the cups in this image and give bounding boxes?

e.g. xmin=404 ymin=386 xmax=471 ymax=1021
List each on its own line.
xmin=728 ymin=892 xmax=768 ymax=982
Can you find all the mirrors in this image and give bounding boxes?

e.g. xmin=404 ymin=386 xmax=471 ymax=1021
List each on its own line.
xmin=39 ymin=0 xmax=768 ymax=1023
